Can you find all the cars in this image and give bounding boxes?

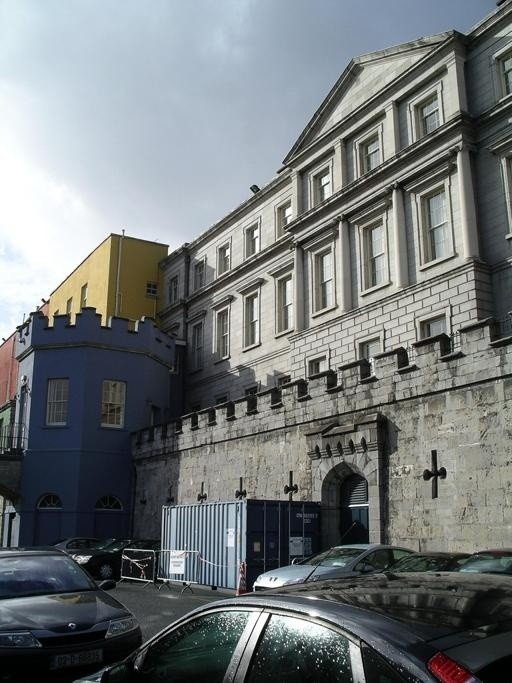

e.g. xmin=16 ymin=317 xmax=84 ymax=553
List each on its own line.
xmin=45 ymin=536 xmax=107 ymax=555
xmin=71 ymin=572 xmax=512 ymax=683
xmin=450 ymin=547 xmax=512 ymax=575
xmin=0 ymin=543 xmax=143 ymax=683
xmin=252 ymin=543 xmax=418 ymax=593
xmin=380 ymin=550 xmax=472 ymax=572
xmin=71 ymin=537 xmax=161 ymax=580
